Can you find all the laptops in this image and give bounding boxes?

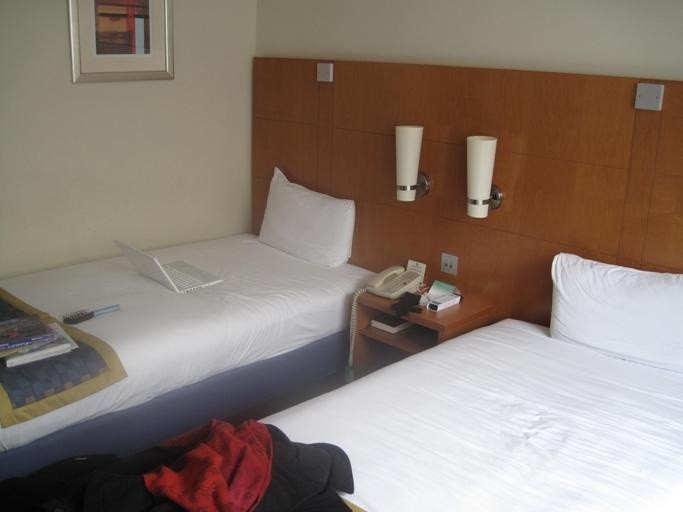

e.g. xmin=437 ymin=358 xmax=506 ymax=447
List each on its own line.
xmin=112 ymin=238 xmax=223 ymax=295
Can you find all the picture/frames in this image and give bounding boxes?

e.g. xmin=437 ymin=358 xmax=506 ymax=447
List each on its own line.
xmin=67 ymin=0 xmax=174 ymax=85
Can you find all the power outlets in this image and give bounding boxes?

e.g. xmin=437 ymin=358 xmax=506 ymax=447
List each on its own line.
xmin=439 ymin=252 xmax=458 ymax=277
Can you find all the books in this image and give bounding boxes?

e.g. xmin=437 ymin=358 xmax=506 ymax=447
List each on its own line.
xmin=0 ymin=312 xmax=56 ymax=358
xmin=3 ymin=322 xmax=78 ymax=368
xmin=370 ymin=322 xmax=414 ymax=335
xmin=370 ymin=314 xmax=411 ymax=330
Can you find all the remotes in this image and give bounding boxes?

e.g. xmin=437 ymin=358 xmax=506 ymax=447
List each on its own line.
xmin=427 ymin=294 xmax=460 ymax=311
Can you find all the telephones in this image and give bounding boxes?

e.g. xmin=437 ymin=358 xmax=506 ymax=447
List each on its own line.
xmin=366 ymin=266 xmax=422 ymax=299
